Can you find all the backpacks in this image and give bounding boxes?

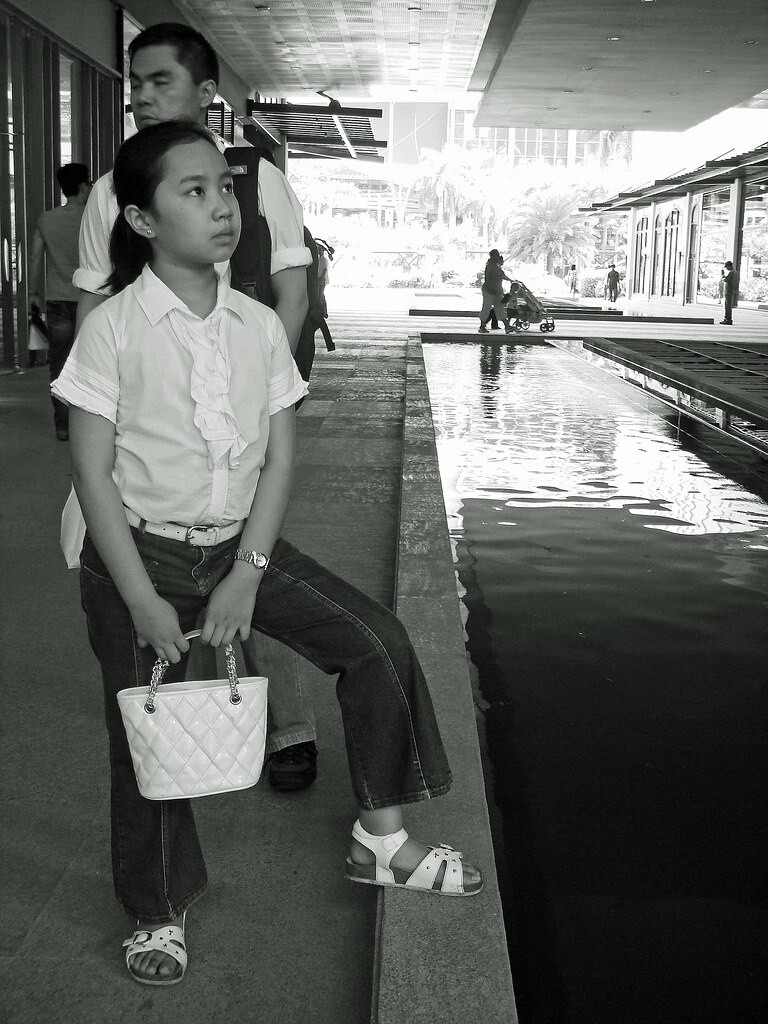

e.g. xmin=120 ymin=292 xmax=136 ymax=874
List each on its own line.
xmin=223 ymin=146 xmax=336 ymax=412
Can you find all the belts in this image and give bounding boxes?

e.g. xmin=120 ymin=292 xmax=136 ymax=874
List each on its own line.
xmin=123 ymin=506 xmax=246 ymax=548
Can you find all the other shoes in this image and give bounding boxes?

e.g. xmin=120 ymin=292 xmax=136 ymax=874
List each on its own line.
xmin=491 ymin=326 xmax=501 ymax=329
xmin=56 ymin=428 xmax=69 ymax=442
xmin=719 ymin=319 xmax=732 ymax=325
xmin=478 ymin=327 xmax=490 ymax=333
xmin=505 ymin=326 xmax=517 ymax=334
xmin=263 ymin=739 xmax=318 ymax=793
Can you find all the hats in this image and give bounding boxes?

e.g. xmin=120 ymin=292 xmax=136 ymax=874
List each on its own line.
xmin=724 ymin=261 xmax=734 ymax=267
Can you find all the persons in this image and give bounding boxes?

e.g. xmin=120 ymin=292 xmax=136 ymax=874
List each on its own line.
xmin=49 ymin=118 xmax=486 ymax=986
xmin=603 ymin=264 xmax=621 ymax=302
xmin=22 ymin=20 xmax=328 ymax=794
xmin=567 ymin=264 xmax=577 ymax=298
xmin=476 ymin=248 xmax=515 ymax=334
xmin=718 ymin=261 xmax=737 ymax=325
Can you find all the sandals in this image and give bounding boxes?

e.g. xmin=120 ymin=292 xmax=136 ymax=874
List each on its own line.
xmin=345 ymin=819 xmax=484 ymax=896
xmin=122 ymin=910 xmax=187 ymax=986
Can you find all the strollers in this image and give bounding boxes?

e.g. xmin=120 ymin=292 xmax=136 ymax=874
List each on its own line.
xmin=511 ymin=279 xmax=555 ymax=332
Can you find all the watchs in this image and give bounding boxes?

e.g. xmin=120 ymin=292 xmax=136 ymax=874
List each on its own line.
xmin=232 ymin=546 xmax=270 ymax=572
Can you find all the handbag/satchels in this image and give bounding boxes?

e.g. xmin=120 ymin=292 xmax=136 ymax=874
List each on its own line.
xmin=117 ymin=630 xmax=269 ymax=800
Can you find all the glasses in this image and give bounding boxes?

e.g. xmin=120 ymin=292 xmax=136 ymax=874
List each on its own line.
xmin=85 ymin=180 xmax=94 ymax=188
